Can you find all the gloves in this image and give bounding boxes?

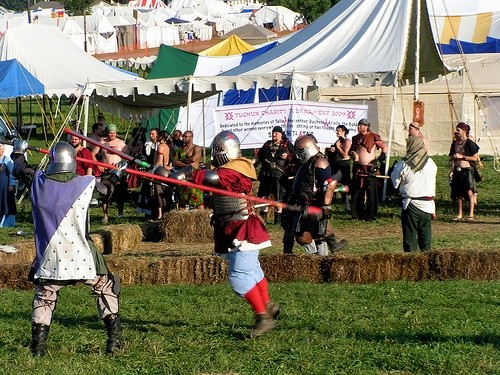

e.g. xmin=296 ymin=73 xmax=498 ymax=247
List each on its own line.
xmin=10 ymin=139 xmax=29 ymax=159
xmin=109 ymin=162 xmax=127 ymax=180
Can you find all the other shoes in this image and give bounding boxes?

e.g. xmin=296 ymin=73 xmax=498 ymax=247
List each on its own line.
xmin=267 ymin=300 xmax=280 ymax=317
xmin=250 ymin=312 xmax=280 ymax=337
xmin=453 ymin=216 xmax=474 ymax=221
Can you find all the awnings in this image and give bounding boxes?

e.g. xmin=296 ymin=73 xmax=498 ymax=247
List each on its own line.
xmin=131 ymin=34 xmax=282 ymax=167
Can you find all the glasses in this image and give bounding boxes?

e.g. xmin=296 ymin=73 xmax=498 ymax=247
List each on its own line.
xmin=182 ymin=136 xmax=192 ymax=138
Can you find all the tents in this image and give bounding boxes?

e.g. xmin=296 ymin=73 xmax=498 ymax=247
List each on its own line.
xmin=0 ymin=24 xmax=145 ymax=148
xmin=173 ymin=0 xmax=304 ymax=43
xmin=85 ymin=0 xmax=192 ymax=54
xmin=82 ymin=1 xmax=500 ymax=224
xmin=0 ymin=6 xmax=96 ymax=54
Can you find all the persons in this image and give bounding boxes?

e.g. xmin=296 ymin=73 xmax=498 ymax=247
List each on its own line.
xmin=406 ymin=122 xmax=429 ymax=156
xmin=138 ymin=130 xmax=282 ymax=337
xmin=326 ymin=118 xmax=388 ymax=222
xmin=10 ymin=138 xmax=129 ymax=358
xmin=448 ymin=122 xmax=484 ymax=220
xmin=139 ymin=128 xmax=202 ymax=220
xmin=391 ymin=136 xmax=438 ymax=253
xmin=253 ymin=126 xmax=349 ymax=256
xmin=68 ymin=123 xmax=129 ymax=223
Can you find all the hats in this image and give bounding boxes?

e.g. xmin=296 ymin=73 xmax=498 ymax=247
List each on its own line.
xmin=359 ymin=118 xmax=371 ymax=127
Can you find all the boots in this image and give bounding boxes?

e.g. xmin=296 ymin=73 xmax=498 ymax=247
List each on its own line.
xmin=29 ymin=321 xmax=51 ymax=360
xmin=274 ymin=211 xmax=282 ymax=225
xmin=325 ymin=233 xmax=348 ymax=254
xmin=103 ymin=311 xmax=124 ymax=354
xmin=259 ymin=210 xmax=268 ymax=224
xmin=282 ymin=239 xmax=295 ymax=254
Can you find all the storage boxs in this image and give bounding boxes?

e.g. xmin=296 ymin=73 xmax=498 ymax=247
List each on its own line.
xmin=21 ymin=125 xmax=37 ymax=137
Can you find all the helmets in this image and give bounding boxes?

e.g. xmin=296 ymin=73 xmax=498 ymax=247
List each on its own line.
xmin=210 ymin=130 xmax=242 ymax=167
xmin=294 ymin=135 xmax=320 ymax=164
xmin=46 ymin=141 xmax=77 ymax=175
